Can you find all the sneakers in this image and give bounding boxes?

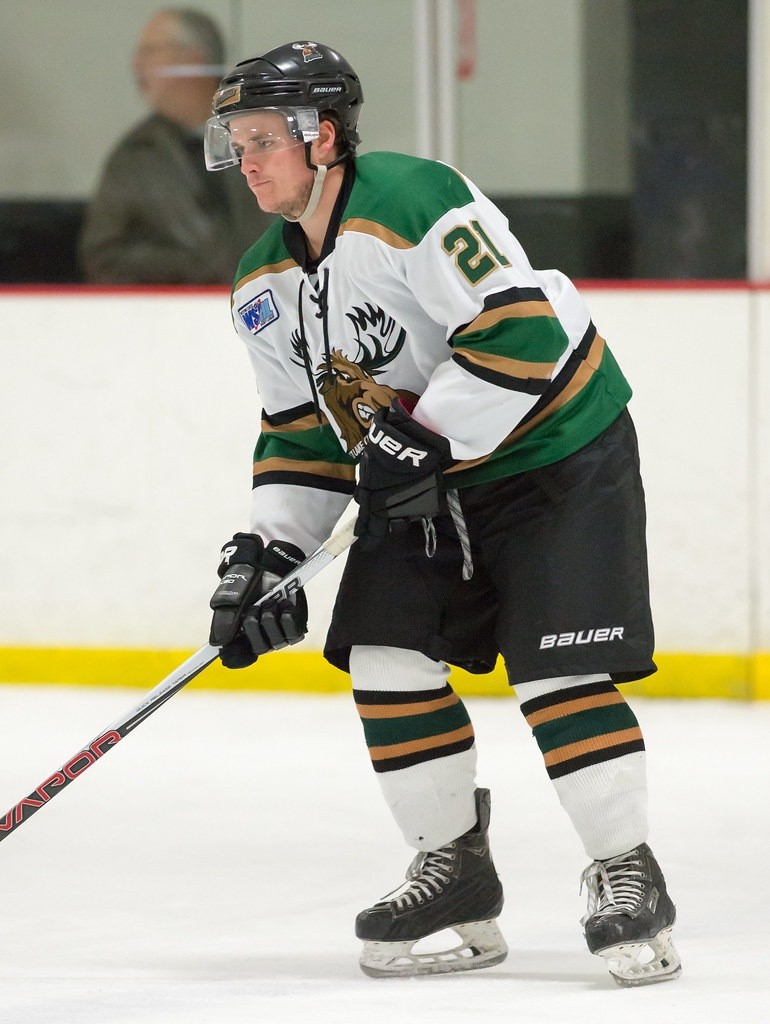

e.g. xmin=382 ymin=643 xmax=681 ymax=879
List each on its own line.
xmin=579 ymin=841 xmax=683 ymax=987
xmin=355 ymin=788 xmax=509 ymax=979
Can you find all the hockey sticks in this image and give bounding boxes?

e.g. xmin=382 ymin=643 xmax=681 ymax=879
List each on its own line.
xmin=0 ymin=515 xmax=359 ymax=843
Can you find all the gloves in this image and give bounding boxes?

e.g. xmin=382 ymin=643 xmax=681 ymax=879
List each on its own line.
xmin=209 ymin=531 xmax=309 ymax=669
xmin=352 ymin=395 xmax=463 ymax=537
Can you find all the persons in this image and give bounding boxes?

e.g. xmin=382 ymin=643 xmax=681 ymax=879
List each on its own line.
xmin=206 ymin=37 xmax=685 ymax=986
xmin=82 ymin=8 xmax=284 ymax=287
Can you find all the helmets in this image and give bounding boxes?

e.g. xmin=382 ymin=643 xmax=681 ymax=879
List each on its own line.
xmin=212 ymin=39 xmax=364 ymax=170
